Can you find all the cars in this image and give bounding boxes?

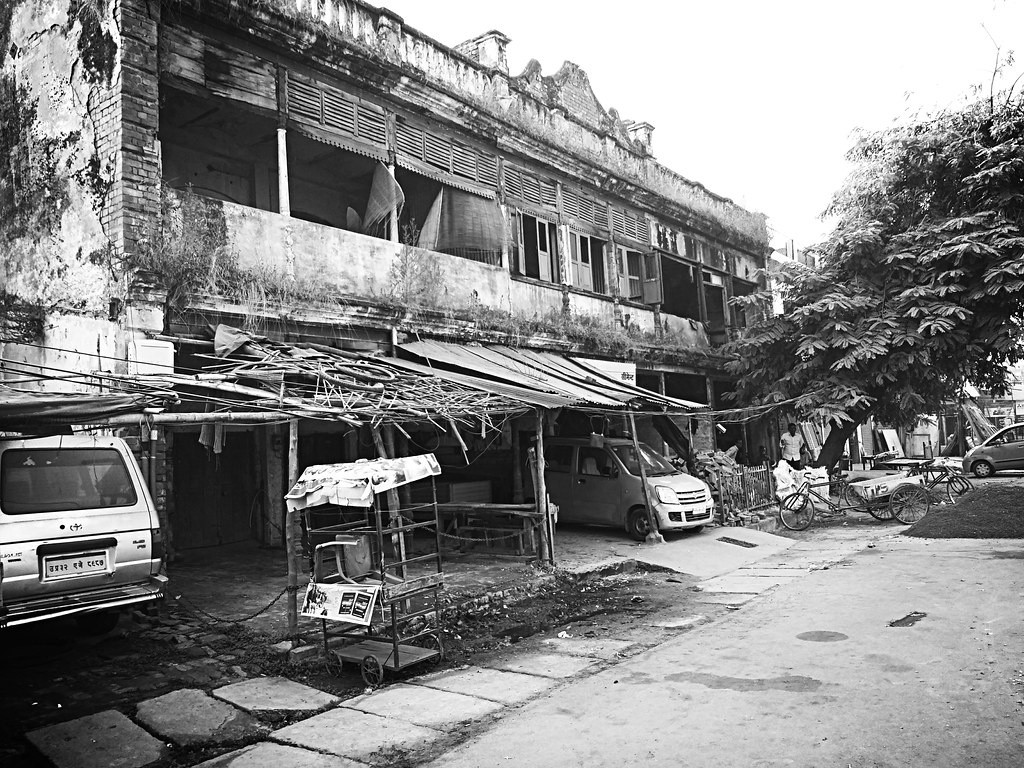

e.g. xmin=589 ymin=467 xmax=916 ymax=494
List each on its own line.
xmin=962 ymin=423 xmax=1024 ymax=478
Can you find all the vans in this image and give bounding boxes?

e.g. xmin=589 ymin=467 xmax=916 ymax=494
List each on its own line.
xmin=525 ymin=437 xmax=715 ymax=542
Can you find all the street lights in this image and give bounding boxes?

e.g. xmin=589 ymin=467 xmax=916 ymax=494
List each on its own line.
xmin=1011 ymin=388 xmax=1022 ymax=441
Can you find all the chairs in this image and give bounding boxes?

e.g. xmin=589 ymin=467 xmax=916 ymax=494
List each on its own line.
xmin=581 ymin=456 xmax=601 ymax=475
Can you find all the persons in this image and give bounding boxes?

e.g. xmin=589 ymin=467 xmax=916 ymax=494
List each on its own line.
xmin=779 ymin=423 xmax=803 ymax=472
xmin=726 ymin=438 xmax=743 ymax=462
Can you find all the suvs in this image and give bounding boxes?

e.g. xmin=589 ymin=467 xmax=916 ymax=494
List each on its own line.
xmin=0 ymin=433 xmax=168 ymax=636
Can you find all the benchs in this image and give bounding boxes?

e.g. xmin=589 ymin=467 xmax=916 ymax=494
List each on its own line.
xmin=457 ymin=522 xmax=527 ymax=554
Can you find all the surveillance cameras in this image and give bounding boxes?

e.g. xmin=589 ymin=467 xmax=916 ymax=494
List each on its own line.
xmin=716 ymin=424 xmax=727 ymax=433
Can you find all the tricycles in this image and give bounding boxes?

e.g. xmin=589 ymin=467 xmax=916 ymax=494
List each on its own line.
xmin=779 ymin=457 xmax=976 ymax=531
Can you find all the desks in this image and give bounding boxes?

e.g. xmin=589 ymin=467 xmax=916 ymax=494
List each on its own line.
xmin=401 ymin=501 xmax=557 ymax=566
xmin=861 ymin=456 xmax=875 ymax=470
xmin=878 ymin=457 xmax=932 ymax=473
